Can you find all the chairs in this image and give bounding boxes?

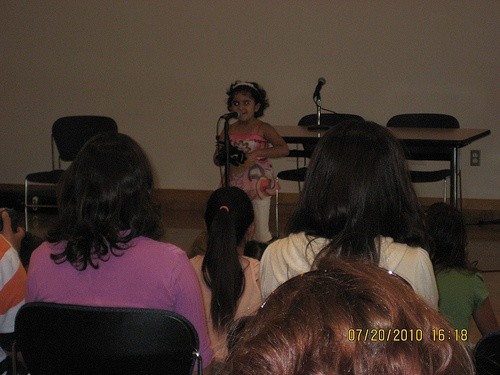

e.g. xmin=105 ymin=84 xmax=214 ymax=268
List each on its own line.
xmin=23 ymin=115 xmax=118 ymax=232
xmin=387 ymin=114 xmax=460 ymax=205
xmin=11 ymin=302 xmax=203 ymax=375
xmin=274 ymin=113 xmax=365 ymax=238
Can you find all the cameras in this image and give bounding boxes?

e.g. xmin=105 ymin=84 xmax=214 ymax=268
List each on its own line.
xmin=0 ymin=207 xmax=17 ymax=233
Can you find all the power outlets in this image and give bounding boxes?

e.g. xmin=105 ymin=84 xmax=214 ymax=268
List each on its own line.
xmin=470 ymin=149 xmax=482 ymax=166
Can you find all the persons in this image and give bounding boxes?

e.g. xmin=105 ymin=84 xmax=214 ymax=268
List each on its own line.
xmin=423 ymin=201 xmax=500 ymax=349
xmin=188 ymin=186 xmax=261 ymax=362
xmin=0 ymin=212 xmax=28 ymax=375
xmin=25 ymin=132 xmax=215 ymax=375
xmin=260 ymin=120 xmax=439 ymax=309
xmin=213 ymin=82 xmax=289 ymax=244
xmin=218 ymin=262 xmax=476 ymax=375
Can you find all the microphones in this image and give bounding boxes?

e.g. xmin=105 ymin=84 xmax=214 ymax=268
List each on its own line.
xmin=313 ymin=78 xmax=325 ymax=97
xmin=220 ymin=111 xmax=240 ymax=119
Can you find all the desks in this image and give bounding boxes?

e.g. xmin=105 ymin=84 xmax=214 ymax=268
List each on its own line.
xmin=271 ymin=124 xmax=491 ymax=209
